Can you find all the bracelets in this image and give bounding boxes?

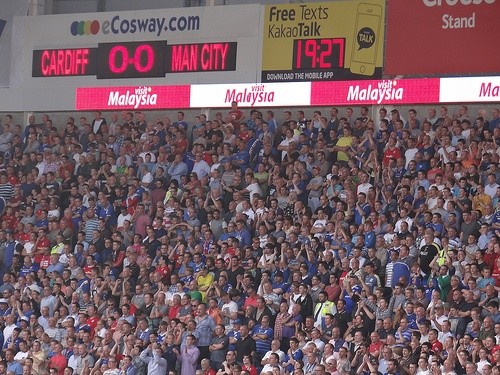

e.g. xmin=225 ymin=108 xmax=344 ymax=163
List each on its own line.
xmin=433 ymin=318 xmax=435 ymax=321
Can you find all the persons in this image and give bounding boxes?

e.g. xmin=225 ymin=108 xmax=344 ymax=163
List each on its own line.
xmin=0 ymin=101 xmax=500 ymax=375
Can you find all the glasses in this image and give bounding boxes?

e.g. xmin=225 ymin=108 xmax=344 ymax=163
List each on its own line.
xmin=191 ymin=305 xmax=196 ymax=307
xmin=109 ymin=304 xmax=114 ymax=306
xmin=328 ymin=363 xmax=332 ymax=365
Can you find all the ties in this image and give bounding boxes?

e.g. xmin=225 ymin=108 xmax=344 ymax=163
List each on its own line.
xmin=314 ymin=302 xmax=323 ymax=322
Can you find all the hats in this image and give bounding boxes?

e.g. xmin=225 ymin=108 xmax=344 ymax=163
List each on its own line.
xmin=18 ymin=316 xmax=29 ymax=323
xmin=0 ymin=298 xmax=8 ymax=303
xmin=369 ymin=357 xmax=379 ymax=365
